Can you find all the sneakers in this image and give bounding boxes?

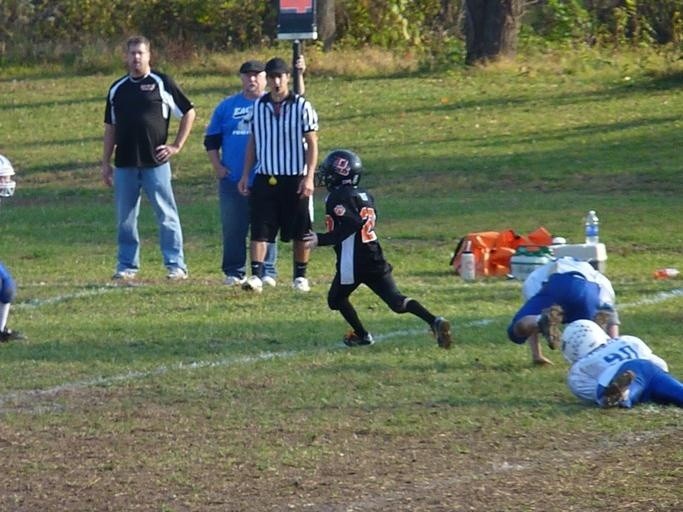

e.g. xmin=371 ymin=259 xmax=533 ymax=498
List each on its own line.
xmin=261 ymin=276 xmax=277 ymax=287
xmin=294 ymin=277 xmax=311 ymax=292
xmin=222 ymin=276 xmax=245 ymax=286
xmin=0 ymin=327 xmax=29 ymax=343
xmin=342 ymin=332 xmax=374 ymax=346
xmin=166 ymin=269 xmax=189 ymax=280
xmin=433 ymin=316 xmax=452 ymax=348
xmin=112 ymin=271 xmax=135 ymax=280
xmin=538 ymin=305 xmax=563 ymax=350
xmin=242 ymin=275 xmax=262 ymax=291
xmin=605 ymin=369 xmax=636 ymax=408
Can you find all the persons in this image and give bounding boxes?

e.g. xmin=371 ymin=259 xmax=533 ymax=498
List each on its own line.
xmin=0 ymin=154 xmax=28 ymax=342
xmin=302 ymin=151 xmax=453 ymax=350
xmin=506 ymin=255 xmax=620 ymax=365
xmin=238 ymin=56 xmax=319 ymax=293
xmin=205 ymin=55 xmax=307 ymax=284
xmin=102 ymin=35 xmax=195 ymax=281
xmin=559 ymin=319 xmax=682 ymax=415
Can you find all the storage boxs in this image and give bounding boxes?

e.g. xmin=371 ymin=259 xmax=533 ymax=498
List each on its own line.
xmin=510 ymin=256 xmax=548 ymax=279
xmin=549 ymin=243 xmax=608 ymax=273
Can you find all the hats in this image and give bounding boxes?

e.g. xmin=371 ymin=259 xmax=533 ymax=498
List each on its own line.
xmin=264 ymin=58 xmax=289 ymax=75
xmin=240 ymin=60 xmax=264 ymax=73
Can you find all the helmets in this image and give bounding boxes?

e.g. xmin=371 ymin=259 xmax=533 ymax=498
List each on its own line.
xmin=0 ymin=155 xmax=16 ymax=197
xmin=314 ymin=149 xmax=361 ymax=190
xmin=561 ymin=320 xmax=607 ymax=362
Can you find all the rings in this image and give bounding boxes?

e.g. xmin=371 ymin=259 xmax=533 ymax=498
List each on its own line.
xmin=164 ymin=151 xmax=169 ymax=155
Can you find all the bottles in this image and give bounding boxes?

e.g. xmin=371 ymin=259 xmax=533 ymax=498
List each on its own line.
xmin=460 ymin=251 xmax=476 ymax=282
xmin=584 ymin=210 xmax=599 ymax=243
xmin=653 ymin=268 xmax=680 ymax=281
